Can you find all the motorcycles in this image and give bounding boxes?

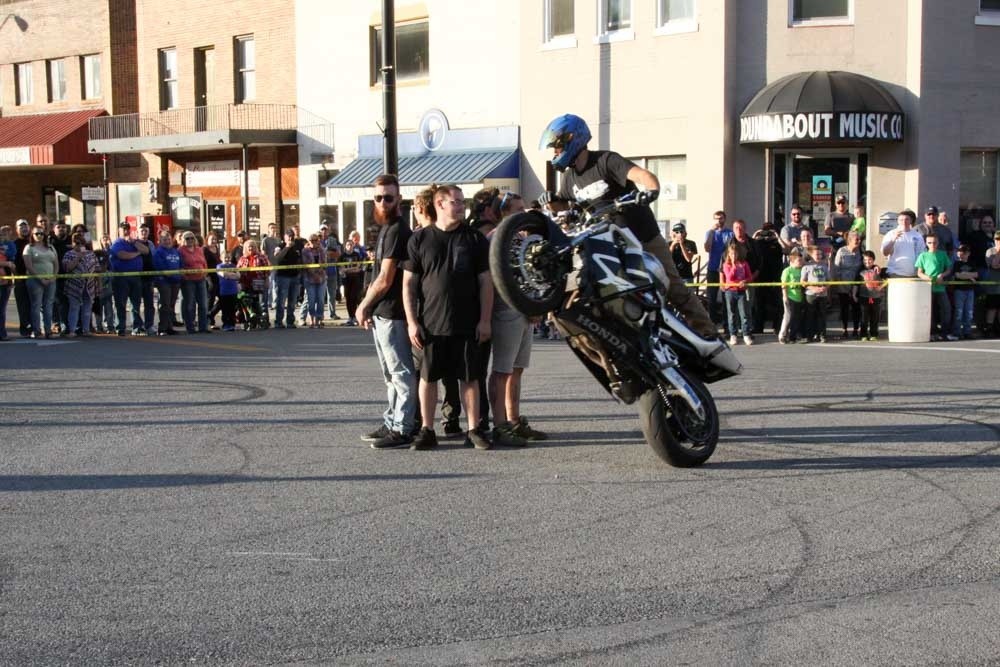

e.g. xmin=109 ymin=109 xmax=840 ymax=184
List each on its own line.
xmin=487 ymin=190 xmax=745 ymax=468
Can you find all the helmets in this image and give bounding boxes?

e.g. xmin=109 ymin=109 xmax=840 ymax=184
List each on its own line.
xmin=538 ymin=114 xmax=592 ymax=173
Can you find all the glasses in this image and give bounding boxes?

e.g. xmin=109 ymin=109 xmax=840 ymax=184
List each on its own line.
xmin=74 ymin=241 xmax=85 ymax=245
xmin=185 ymin=237 xmax=194 ymax=240
xmin=499 ymin=192 xmax=513 ymax=210
xmin=791 ymin=212 xmax=801 ymax=215
xmin=443 ymin=199 xmax=466 ymax=206
xmin=33 ymin=232 xmax=44 ymax=235
xmin=675 ymin=231 xmax=684 ymax=234
xmin=374 ymin=194 xmax=396 ymax=203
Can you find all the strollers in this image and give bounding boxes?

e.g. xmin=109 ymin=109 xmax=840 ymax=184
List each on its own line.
xmin=237 ymin=289 xmax=271 ymax=330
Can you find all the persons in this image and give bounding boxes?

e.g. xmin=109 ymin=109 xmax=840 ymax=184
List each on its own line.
xmin=667 ymin=223 xmax=698 ymax=283
xmin=704 ymin=193 xmax=882 ymax=345
xmin=355 ymin=175 xmax=416 ymax=450
xmin=402 ymin=182 xmax=548 ymax=449
xmin=12 ymin=213 xmax=270 ymax=339
xmin=879 ymin=205 xmax=1000 ymax=341
xmin=536 ymin=114 xmax=718 ymax=340
xmin=0 ymin=225 xmax=17 ymax=341
xmin=261 ymin=223 xmax=375 ymax=329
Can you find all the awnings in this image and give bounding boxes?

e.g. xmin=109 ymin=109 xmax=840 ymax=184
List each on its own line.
xmin=739 ymin=70 xmax=904 ymax=146
xmin=0 ymin=109 xmax=108 ymax=165
xmin=321 ymin=151 xmax=520 ymax=202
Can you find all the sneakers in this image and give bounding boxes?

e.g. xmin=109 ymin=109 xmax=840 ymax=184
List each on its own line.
xmin=465 ymin=429 xmax=492 ymax=449
xmin=508 ymin=415 xmax=548 ymax=440
xmin=442 ymin=425 xmax=462 ymax=438
xmin=361 ymin=426 xmax=390 ymax=441
xmin=409 ymin=428 xmax=437 ymax=450
xmin=491 ymin=424 xmax=528 ymax=446
xmin=371 ymin=429 xmax=415 ymax=449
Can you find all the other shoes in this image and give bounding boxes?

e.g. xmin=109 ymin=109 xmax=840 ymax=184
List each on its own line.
xmin=932 ymin=328 xmax=1000 ymax=342
xmin=3 ymin=319 xmax=370 ymax=337
xmin=716 ymin=322 xmax=879 ymax=346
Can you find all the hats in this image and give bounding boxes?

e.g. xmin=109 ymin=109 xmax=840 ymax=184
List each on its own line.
xmin=925 ymin=207 xmax=938 ymax=214
xmin=16 ymin=219 xmax=28 ymax=226
xmin=319 ymin=224 xmax=328 ymax=230
xmin=673 ymin=224 xmax=684 ymax=231
xmin=836 ymin=195 xmax=846 ymax=203
xmin=237 ymin=230 xmax=245 ymax=237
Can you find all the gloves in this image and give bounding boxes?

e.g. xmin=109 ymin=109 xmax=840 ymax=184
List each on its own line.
xmin=631 ymin=190 xmax=659 ymax=204
xmin=538 ymin=191 xmax=556 ymax=205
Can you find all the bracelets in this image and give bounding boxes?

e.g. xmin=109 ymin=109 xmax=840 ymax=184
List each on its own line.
xmin=134 ymin=240 xmax=136 ymax=244
